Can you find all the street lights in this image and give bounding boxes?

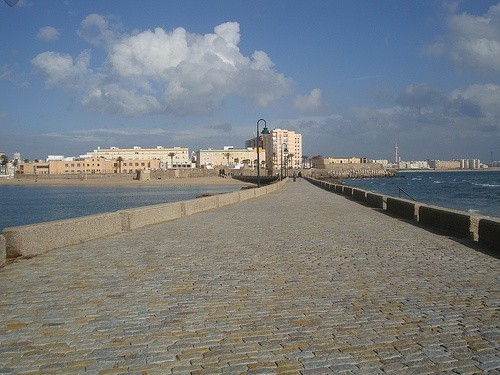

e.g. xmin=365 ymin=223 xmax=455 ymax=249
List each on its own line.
xmin=280 ymin=142 xmax=294 ymax=182
xmin=256 ymin=118 xmax=270 ymax=188
